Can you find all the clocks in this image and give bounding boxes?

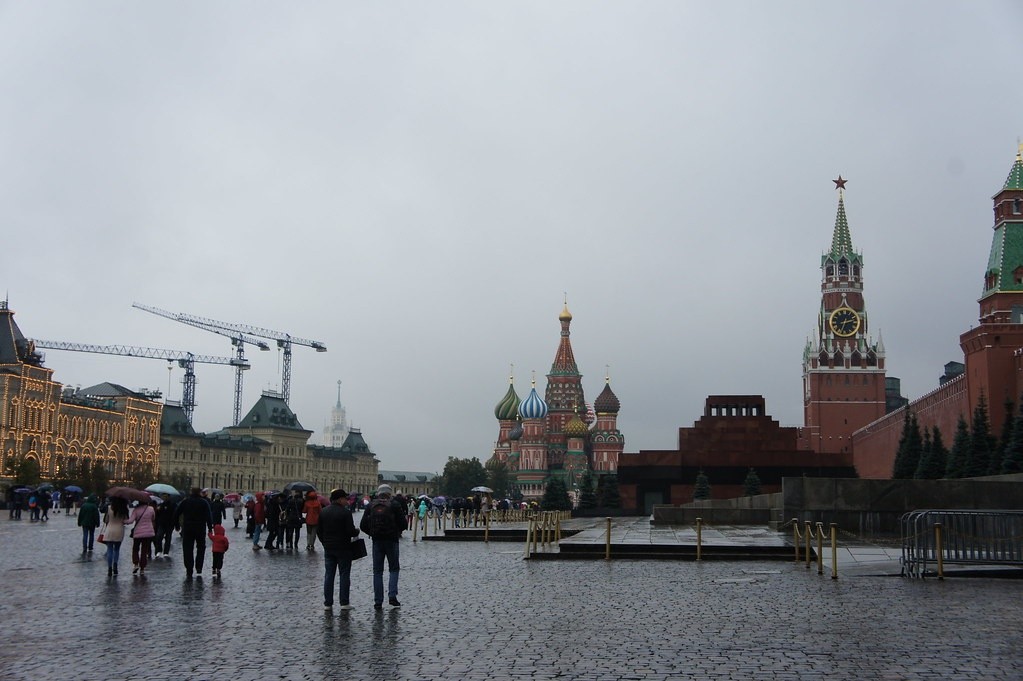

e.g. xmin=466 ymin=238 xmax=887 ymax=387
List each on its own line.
xmin=829 ymin=307 xmax=861 ymax=337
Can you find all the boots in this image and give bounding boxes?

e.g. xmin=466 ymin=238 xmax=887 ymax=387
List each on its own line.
xmin=112 ymin=562 xmax=118 ymax=575
xmin=108 ymin=567 xmax=112 ymax=577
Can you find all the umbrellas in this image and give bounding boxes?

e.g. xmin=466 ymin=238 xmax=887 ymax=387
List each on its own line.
xmin=418 ymin=486 xmax=539 ymax=505
xmin=0 ymin=463 xmax=316 ymax=503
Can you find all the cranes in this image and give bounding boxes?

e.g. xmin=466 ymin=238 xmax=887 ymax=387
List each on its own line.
xmin=24 ymin=337 xmax=251 ymax=427
xmin=133 ymin=301 xmax=269 ymax=426
xmin=179 ymin=313 xmax=328 ymax=405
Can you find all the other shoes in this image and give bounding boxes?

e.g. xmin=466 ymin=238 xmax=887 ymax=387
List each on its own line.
xmin=155 ymin=552 xmax=161 ymax=558
xmin=140 ymin=569 xmax=144 ymax=575
xmin=164 ymin=554 xmax=168 ymax=557
xmin=325 ymin=604 xmax=332 ymax=611
xmin=341 ymin=606 xmax=355 ymax=610
xmin=374 ymin=602 xmax=382 ymax=610
xmin=389 ymin=598 xmax=401 ymax=606
xmin=252 ymin=541 xmax=314 ymax=551
xmin=132 ymin=565 xmax=139 ymax=573
xmin=212 ymin=571 xmax=216 ymax=575
xmin=217 ymin=570 xmax=221 ymax=577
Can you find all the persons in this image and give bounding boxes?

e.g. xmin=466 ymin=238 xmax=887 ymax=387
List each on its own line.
xmin=360 ymin=485 xmax=408 ymax=606
xmin=0 ymin=492 xmax=536 ymax=575
xmin=174 ymin=488 xmax=212 ymax=576
xmin=316 ymin=489 xmax=359 ymax=611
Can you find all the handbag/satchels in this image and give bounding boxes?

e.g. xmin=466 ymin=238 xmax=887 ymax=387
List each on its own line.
xmin=97 ymin=523 xmax=108 ymax=546
xmin=130 ymin=528 xmax=135 ymax=538
xmin=351 ymin=535 xmax=367 ymax=561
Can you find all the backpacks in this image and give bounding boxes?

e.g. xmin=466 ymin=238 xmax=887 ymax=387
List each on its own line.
xmin=371 ymin=497 xmax=392 ymax=535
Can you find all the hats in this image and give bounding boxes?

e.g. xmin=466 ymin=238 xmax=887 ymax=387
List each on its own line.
xmin=379 ymin=485 xmax=392 ymax=496
xmin=331 ymin=489 xmax=350 ymax=500
